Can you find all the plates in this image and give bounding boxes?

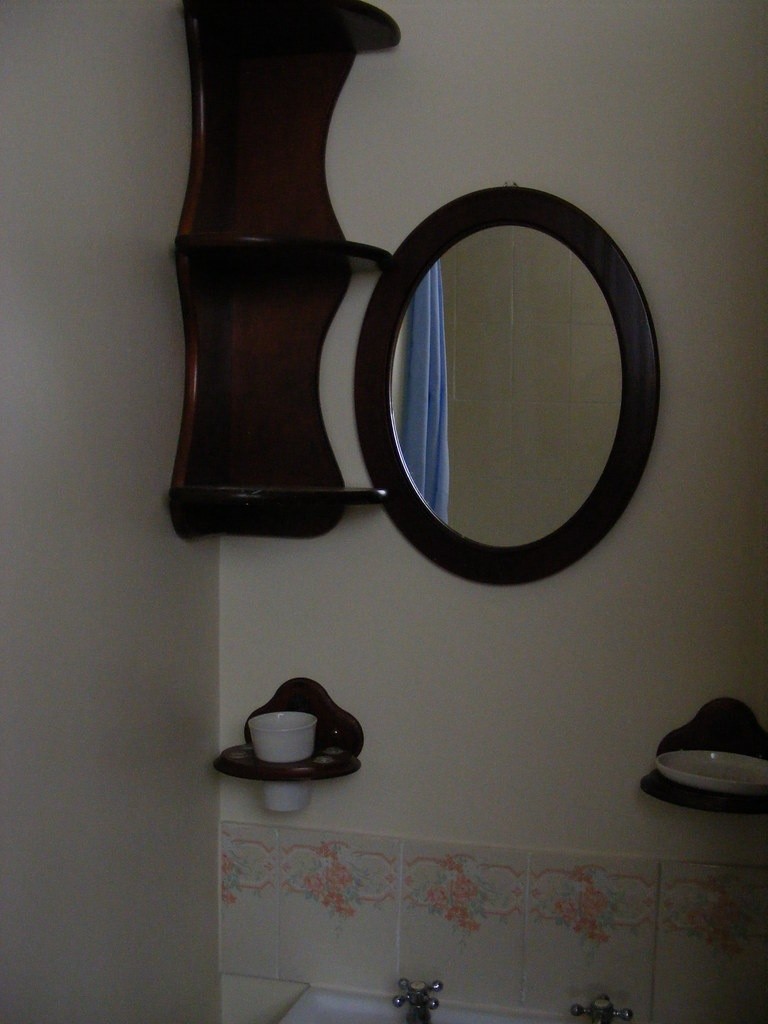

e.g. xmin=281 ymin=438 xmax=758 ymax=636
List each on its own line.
xmin=656 ymin=752 xmax=768 ymax=796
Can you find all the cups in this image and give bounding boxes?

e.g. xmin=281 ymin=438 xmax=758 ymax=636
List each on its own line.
xmin=248 ymin=710 xmax=317 ymax=811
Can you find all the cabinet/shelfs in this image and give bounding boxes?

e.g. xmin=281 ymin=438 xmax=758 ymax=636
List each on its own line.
xmin=166 ymin=0 xmax=403 ymax=543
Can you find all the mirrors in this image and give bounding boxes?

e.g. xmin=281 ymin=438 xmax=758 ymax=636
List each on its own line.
xmin=353 ymin=186 xmax=661 ymax=587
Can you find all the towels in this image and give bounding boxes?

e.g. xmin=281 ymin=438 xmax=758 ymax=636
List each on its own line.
xmin=399 ymin=256 xmax=450 ymax=530
